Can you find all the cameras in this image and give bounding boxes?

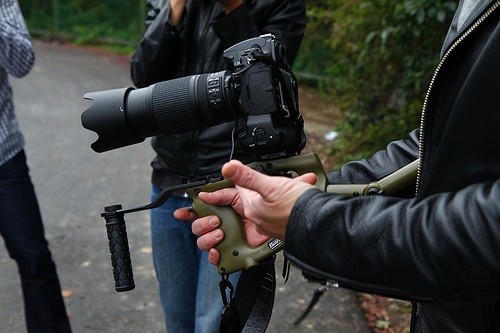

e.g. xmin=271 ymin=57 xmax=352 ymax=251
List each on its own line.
xmin=81 ymin=33 xmax=307 ymax=165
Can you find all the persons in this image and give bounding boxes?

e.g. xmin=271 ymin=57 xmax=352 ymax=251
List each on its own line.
xmin=130 ymin=0 xmax=307 ymax=333
xmin=0 ymin=0 xmax=73 ymax=332
xmin=173 ymin=0 xmax=500 ymax=333
